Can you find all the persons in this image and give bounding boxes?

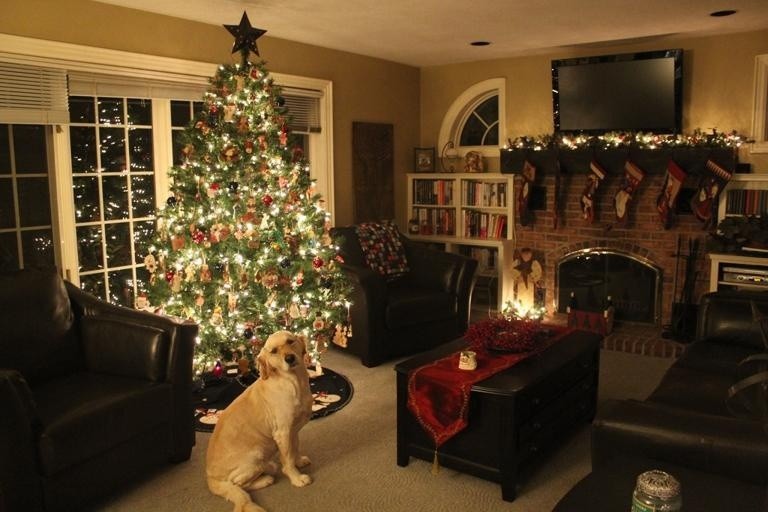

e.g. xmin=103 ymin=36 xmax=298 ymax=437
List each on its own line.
xmin=511 ymin=247 xmax=543 ymax=310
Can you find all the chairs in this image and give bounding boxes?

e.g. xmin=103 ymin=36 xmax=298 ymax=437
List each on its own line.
xmin=327 ymin=223 xmax=480 ymax=369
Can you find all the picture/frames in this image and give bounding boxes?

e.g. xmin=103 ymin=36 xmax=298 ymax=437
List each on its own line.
xmin=412 ymin=144 xmax=437 ymax=174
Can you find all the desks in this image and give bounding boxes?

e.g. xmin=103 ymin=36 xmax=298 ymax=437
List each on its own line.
xmin=392 ymin=326 xmax=608 ymax=503
xmin=549 ymin=466 xmax=768 ymax=512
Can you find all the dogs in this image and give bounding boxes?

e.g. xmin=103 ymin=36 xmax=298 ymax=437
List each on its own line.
xmin=205 ymin=329 xmax=314 ymax=512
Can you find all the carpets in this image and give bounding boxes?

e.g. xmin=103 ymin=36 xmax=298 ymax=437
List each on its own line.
xmin=195 ymin=364 xmax=355 ymax=435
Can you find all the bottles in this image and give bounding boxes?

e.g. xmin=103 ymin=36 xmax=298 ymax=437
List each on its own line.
xmin=409 ymin=218 xmax=432 ymax=236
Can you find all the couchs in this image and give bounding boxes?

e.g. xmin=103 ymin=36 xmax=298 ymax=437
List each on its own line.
xmin=0 ymin=259 xmax=202 ymax=510
xmin=590 ymin=287 xmax=766 ymax=473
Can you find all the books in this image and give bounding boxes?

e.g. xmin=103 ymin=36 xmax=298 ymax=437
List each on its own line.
xmin=727 ymin=190 xmax=768 ymax=217
xmin=741 ymin=241 xmax=768 ymax=253
xmin=414 ymin=179 xmax=508 ymax=308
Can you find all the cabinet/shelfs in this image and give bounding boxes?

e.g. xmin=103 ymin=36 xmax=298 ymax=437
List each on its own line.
xmin=703 ymin=251 xmax=767 ymax=292
xmin=714 ymin=169 xmax=767 ymax=255
xmin=454 ymin=172 xmax=517 ymax=242
xmin=448 ymin=235 xmax=516 ymax=313
xmin=411 ymin=235 xmax=456 ymax=254
xmin=406 ymin=170 xmax=459 ymax=242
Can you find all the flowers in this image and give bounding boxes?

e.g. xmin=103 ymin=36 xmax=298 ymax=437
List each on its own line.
xmin=463 ymin=302 xmax=550 ymax=354
